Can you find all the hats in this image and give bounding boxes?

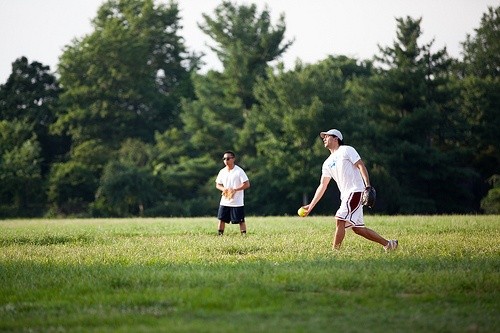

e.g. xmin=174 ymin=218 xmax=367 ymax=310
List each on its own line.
xmin=320 ymin=128 xmax=343 ymax=141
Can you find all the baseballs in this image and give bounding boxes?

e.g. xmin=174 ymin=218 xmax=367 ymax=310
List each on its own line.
xmin=297 ymin=208 xmax=307 ymax=217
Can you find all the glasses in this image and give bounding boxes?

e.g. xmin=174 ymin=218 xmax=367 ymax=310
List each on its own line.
xmin=222 ymin=157 xmax=233 ymax=160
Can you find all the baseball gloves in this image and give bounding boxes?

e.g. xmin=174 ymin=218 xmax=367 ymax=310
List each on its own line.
xmin=362 ymin=187 xmax=376 ymax=208
xmin=223 ymin=188 xmax=235 ymax=201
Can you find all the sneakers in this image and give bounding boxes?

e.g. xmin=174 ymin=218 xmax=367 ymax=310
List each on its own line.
xmin=385 ymin=240 xmax=398 ymax=250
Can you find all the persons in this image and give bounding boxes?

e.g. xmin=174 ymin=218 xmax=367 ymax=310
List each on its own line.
xmin=300 ymin=129 xmax=399 ymax=253
xmin=214 ymin=151 xmax=250 ymax=236
